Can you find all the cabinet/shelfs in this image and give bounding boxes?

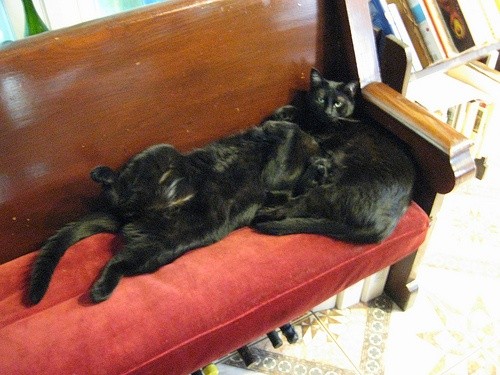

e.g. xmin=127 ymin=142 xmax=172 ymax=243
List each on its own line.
xmin=374 ymin=22 xmax=500 ymax=125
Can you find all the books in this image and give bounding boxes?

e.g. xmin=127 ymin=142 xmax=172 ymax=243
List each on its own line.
xmin=369 ymin=0 xmax=500 ymax=160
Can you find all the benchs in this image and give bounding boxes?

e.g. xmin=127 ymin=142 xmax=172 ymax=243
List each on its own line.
xmin=0 ymin=0 xmax=476 ymax=375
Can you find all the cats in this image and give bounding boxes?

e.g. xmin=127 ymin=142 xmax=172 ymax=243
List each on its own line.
xmin=29 ymin=66 xmax=418 ymax=305
